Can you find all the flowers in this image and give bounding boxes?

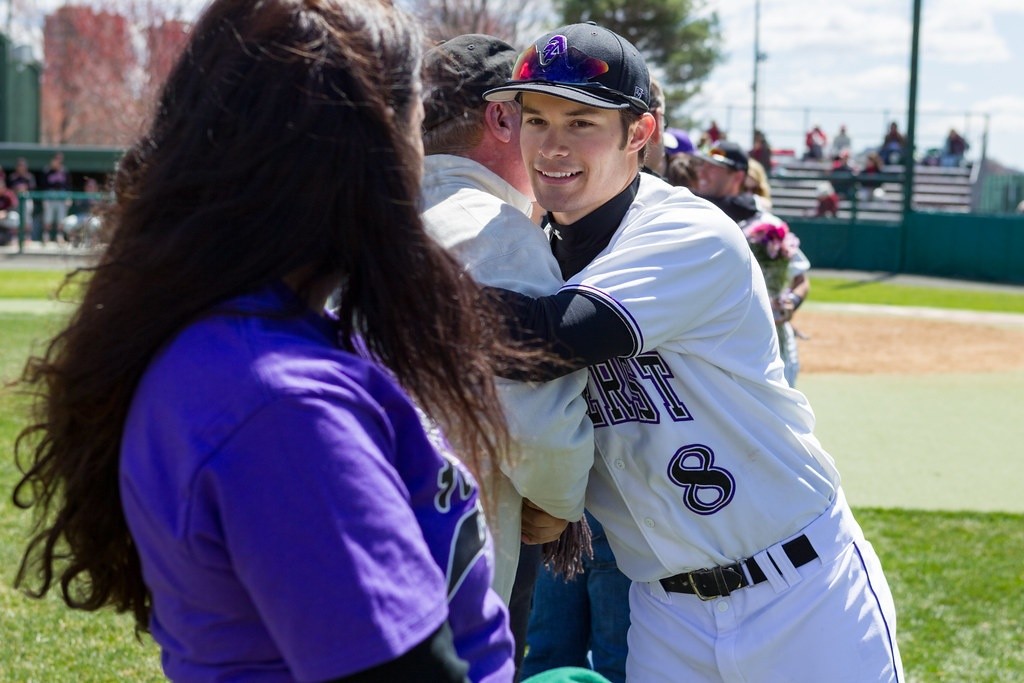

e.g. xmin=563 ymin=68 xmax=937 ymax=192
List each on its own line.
xmin=740 ymin=212 xmax=793 ymax=356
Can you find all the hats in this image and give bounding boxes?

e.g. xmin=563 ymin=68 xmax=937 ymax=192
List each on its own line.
xmin=689 ymin=138 xmax=748 ymax=175
xmin=482 ymin=21 xmax=651 ymax=112
xmin=418 ymin=34 xmax=518 ymax=132
xmin=665 ymin=128 xmax=693 ymax=157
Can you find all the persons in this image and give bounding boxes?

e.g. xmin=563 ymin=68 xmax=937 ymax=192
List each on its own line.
xmin=662 ymin=115 xmax=979 ymax=390
xmin=468 ymin=23 xmax=904 ymax=683
xmin=414 ymin=32 xmax=595 ymax=683
xmin=11 ymin=1 xmax=512 ymax=683
xmin=2 ymin=151 xmax=123 ymax=242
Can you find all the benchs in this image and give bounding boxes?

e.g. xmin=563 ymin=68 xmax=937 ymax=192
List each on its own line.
xmin=770 ymin=157 xmax=971 ymax=219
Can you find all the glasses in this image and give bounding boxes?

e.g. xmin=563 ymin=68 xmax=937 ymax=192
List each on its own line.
xmin=660 ymin=112 xmax=670 ymax=132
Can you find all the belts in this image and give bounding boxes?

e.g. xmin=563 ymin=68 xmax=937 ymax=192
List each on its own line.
xmin=639 ymin=536 xmax=819 ymax=600
